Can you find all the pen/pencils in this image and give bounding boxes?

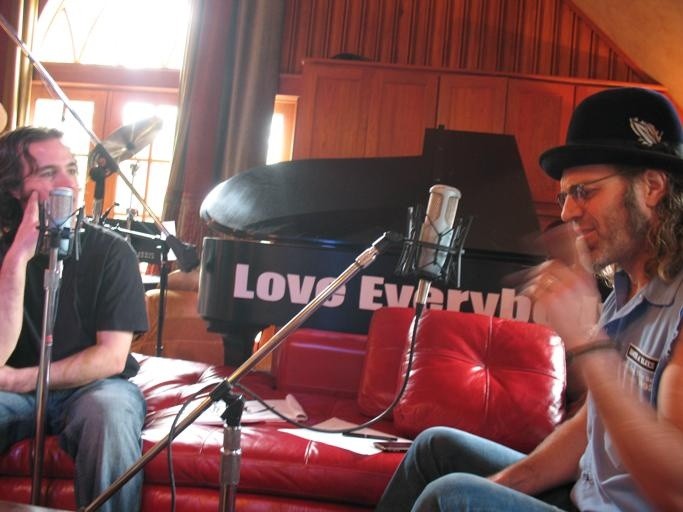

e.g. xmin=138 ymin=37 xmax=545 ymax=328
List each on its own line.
xmin=343 ymin=432 xmax=397 ymax=441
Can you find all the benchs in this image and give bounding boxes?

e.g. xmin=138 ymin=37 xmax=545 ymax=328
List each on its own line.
xmin=0 ymin=327 xmax=414 ymax=510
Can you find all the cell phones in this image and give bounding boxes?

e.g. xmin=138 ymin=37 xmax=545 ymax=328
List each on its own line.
xmin=374 ymin=441 xmax=414 ymax=452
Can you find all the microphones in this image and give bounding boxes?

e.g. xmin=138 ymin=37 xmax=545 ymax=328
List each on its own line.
xmin=46 ymin=188 xmax=73 ymax=245
xmin=415 ymin=184 xmax=463 ymax=320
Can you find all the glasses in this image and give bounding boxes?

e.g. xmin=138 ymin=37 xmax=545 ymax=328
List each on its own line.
xmin=555 ymin=168 xmax=628 ymax=210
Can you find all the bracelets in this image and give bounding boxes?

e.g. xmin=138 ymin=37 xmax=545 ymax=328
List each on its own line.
xmin=567 ymin=338 xmax=618 ymax=363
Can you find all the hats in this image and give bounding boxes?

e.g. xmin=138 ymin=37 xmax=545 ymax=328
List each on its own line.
xmin=539 ymin=87 xmax=682 ymax=181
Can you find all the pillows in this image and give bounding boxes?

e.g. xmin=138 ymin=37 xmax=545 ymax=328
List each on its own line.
xmin=358 ymin=305 xmax=566 ymax=451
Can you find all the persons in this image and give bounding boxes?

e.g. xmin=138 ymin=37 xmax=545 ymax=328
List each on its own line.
xmin=370 ymin=84 xmax=682 ymax=511
xmin=0 ymin=122 xmax=148 ymax=512
